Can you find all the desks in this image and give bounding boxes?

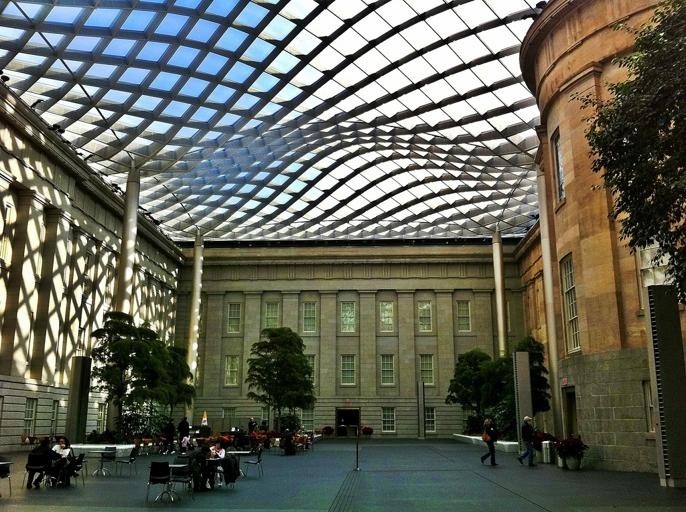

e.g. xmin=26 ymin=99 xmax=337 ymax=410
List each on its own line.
xmin=70 ymin=444 xmax=135 ymax=475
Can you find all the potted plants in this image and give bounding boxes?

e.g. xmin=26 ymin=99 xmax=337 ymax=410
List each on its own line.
xmin=552 ymin=433 xmax=589 ymax=472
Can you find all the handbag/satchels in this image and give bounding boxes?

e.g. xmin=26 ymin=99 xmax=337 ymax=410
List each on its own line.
xmin=482 ymin=430 xmax=492 ymax=442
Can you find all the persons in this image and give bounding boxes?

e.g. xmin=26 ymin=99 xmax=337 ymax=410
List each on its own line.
xmin=25 ymin=414 xmax=269 ymax=489
xmin=481 ymin=417 xmax=500 ymax=465
xmin=517 ymin=415 xmax=537 ymax=466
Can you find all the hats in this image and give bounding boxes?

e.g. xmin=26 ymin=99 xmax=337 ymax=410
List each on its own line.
xmin=523 ymin=415 xmax=532 ymax=422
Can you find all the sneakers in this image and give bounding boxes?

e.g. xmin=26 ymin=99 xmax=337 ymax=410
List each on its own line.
xmin=516 ymin=456 xmax=538 ymax=467
xmin=480 ymin=456 xmax=498 ymax=466
xmin=25 ymin=476 xmax=71 ymax=490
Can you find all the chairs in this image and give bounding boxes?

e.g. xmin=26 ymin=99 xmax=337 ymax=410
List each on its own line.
xmin=144 ymin=445 xmax=262 ymax=505
xmin=21 ymin=452 xmax=87 ymax=490
xmin=92 ymin=446 xmax=141 ymax=478
xmin=0 ymin=456 xmax=13 ymax=498
xmin=198 ymin=427 xmax=316 ymax=452
